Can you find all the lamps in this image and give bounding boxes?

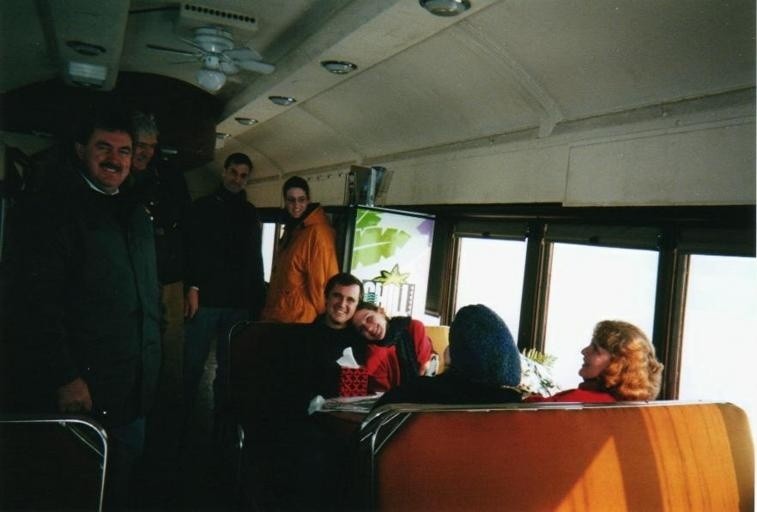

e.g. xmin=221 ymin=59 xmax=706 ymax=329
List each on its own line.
xmin=194 ymin=55 xmax=227 ymax=92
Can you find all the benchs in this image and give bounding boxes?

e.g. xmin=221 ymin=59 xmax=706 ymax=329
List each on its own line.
xmin=0 ymin=412 xmax=113 ymax=512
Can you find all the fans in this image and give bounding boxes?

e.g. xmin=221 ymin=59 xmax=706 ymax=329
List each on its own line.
xmin=145 ymin=28 xmax=276 ymax=75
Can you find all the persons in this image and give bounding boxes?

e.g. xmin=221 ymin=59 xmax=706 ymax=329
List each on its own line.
xmin=372 ymin=304 xmax=522 ymax=408
xmin=520 ymin=321 xmax=665 ymax=404
xmin=0 ymin=112 xmax=438 ymax=512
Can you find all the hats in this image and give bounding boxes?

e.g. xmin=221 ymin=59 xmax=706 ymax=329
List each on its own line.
xmin=450 ymin=304 xmax=521 ymax=385
